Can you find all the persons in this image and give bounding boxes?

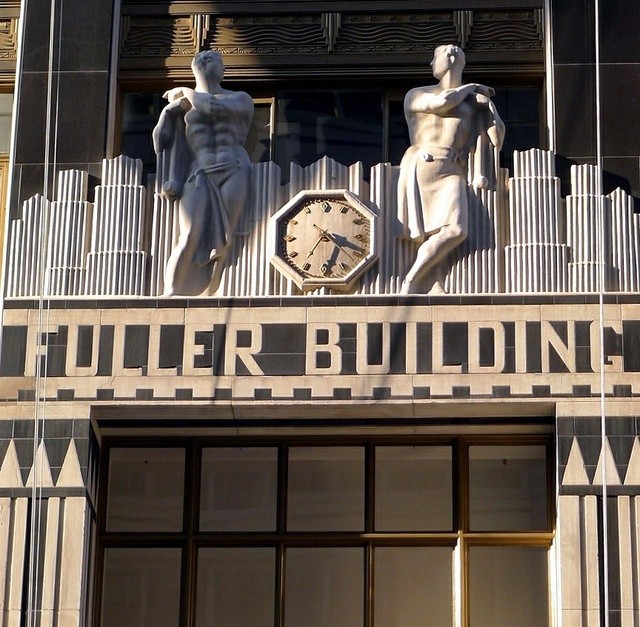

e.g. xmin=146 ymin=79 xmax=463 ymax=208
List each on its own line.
xmin=398 ymin=43 xmax=506 ymax=296
xmin=153 ymin=48 xmax=256 ymax=299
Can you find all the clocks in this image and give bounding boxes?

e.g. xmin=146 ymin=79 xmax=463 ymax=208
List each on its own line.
xmin=268 ymin=190 xmax=379 ymax=289
